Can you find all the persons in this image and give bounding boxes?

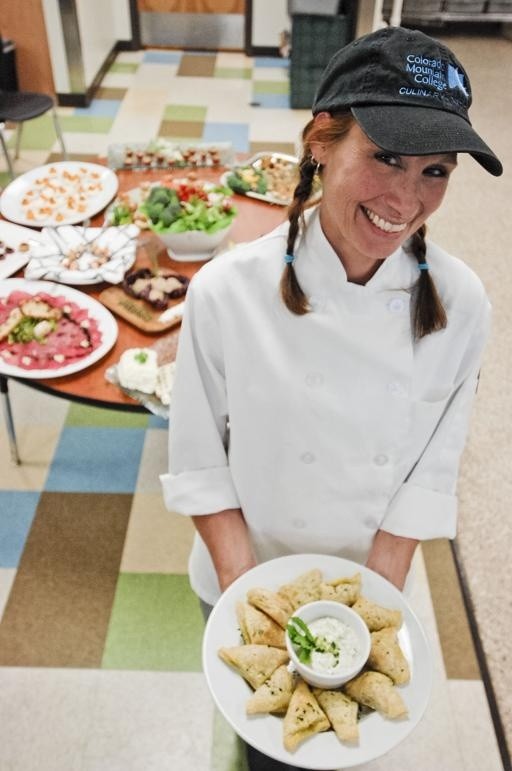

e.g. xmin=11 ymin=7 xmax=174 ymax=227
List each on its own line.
xmin=160 ymin=28 xmax=504 ymax=622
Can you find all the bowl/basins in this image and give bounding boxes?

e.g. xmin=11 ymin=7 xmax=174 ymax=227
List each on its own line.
xmin=151 ymin=212 xmax=233 ymax=263
xmin=282 ymin=598 xmax=373 ymax=693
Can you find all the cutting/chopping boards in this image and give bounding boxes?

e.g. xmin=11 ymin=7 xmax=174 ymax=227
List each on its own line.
xmin=100 ymin=265 xmax=189 ymax=336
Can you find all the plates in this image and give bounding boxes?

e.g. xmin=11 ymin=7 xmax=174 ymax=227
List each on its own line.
xmin=0 ymin=158 xmax=137 ymax=379
xmin=202 ymin=551 xmax=437 ymax=770
xmin=104 ymin=178 xmax=216 ymax=228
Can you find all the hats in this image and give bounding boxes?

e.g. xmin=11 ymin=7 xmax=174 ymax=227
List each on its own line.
xmin=311 ymin=24 xmax=505 ymax=177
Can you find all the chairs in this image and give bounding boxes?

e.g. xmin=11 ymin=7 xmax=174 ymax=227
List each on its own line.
xmin=0 ymin=34 xmax=69 ymax=181
